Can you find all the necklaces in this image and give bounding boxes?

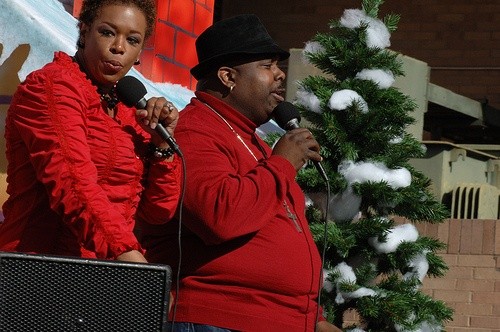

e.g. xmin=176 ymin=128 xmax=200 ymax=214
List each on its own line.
xmin=195 ymin=93 xmax=303 ymax=235
xmin=73 ymin=54 xmax=117 ymax=110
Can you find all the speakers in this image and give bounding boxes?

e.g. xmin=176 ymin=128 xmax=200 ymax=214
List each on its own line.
xmin=0 ymin=252 xmax=171 ymax=332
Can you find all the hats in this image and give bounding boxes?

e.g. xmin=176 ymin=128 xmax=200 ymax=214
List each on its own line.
xmin=190 ymin=13 xmax=290 ymax=81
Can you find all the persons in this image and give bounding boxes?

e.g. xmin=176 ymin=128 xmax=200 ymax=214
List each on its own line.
xmin=166 ymin=18 xmax=342 ymax=332
xmin=0 ymin=1 xmax=181 ymax=266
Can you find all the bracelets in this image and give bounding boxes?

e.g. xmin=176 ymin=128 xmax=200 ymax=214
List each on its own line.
xmin=149 ymin=141 xmax=174 ymax=158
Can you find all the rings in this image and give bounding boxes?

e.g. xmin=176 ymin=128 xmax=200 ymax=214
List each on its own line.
xmin=163 ymin=101 xmax=174 ymax=112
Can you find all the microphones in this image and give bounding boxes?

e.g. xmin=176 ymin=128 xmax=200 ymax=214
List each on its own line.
xmin=116 ymin=76 xmax=183 ymax=157
xmin=273 ymin=102 xmax=328 ymax=181
